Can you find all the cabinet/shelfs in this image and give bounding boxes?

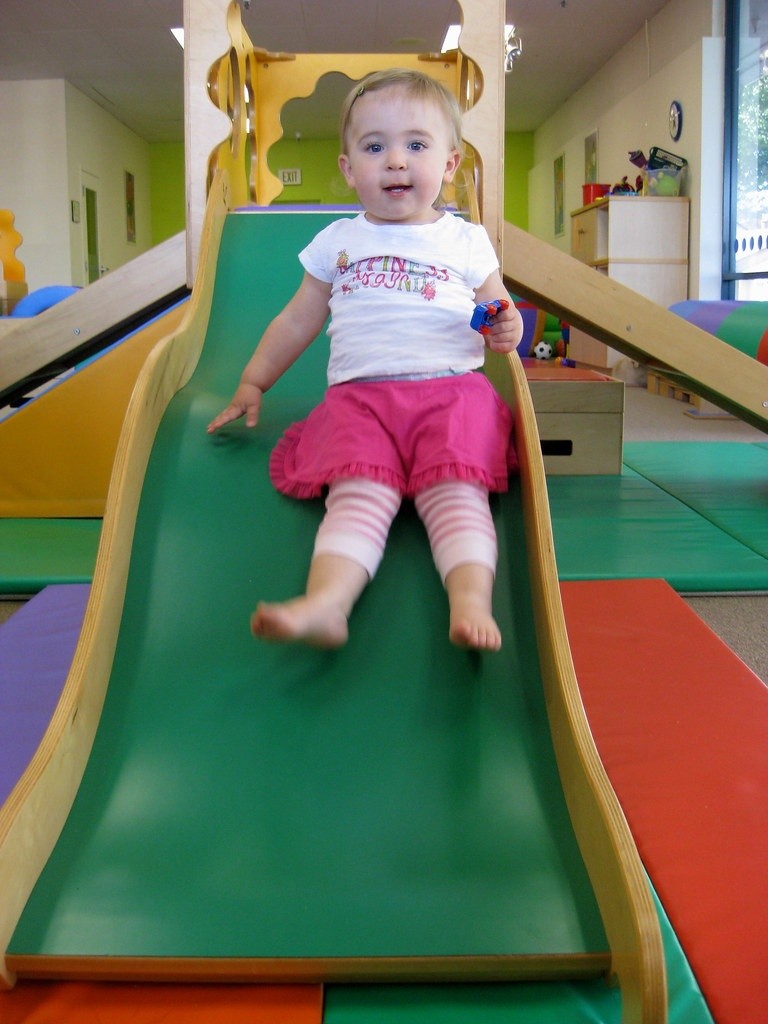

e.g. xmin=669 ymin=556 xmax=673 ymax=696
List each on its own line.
xmin=569 ymin=195 xmax=690 ymax=388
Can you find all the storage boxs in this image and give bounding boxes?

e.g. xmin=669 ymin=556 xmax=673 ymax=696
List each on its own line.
xmin=583 ymin=183 xmax=611 ymax=206
xmin=640 ymin=169 xmax=684 ymax=196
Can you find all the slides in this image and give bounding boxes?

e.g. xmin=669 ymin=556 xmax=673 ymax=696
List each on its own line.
xmin=0 ymin=208 xmax=672 ymax=1024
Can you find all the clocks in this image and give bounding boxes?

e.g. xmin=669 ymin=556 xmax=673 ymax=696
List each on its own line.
xmin=668 ymin=101 xmax=682 ymax=142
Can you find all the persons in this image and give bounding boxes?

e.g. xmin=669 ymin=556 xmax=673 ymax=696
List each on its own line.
xmin=206 ymin=70 xmax=523 ymax=651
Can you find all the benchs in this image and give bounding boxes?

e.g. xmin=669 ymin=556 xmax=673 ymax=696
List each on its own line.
xmin=524 ymin=370 xmax=624 ymax=475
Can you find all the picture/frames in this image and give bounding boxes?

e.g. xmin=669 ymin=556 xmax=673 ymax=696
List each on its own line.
xmin=584 ymin=127 xmax=599 ymax=186
xmin=123 ymin=168 xmax=138 ymax=247
xmin=553 ymin=152 xmax=567 ymax=239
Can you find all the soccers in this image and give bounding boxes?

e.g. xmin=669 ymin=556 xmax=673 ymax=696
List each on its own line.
xmin=533 ymin=341 xmax=553 ymax=360
